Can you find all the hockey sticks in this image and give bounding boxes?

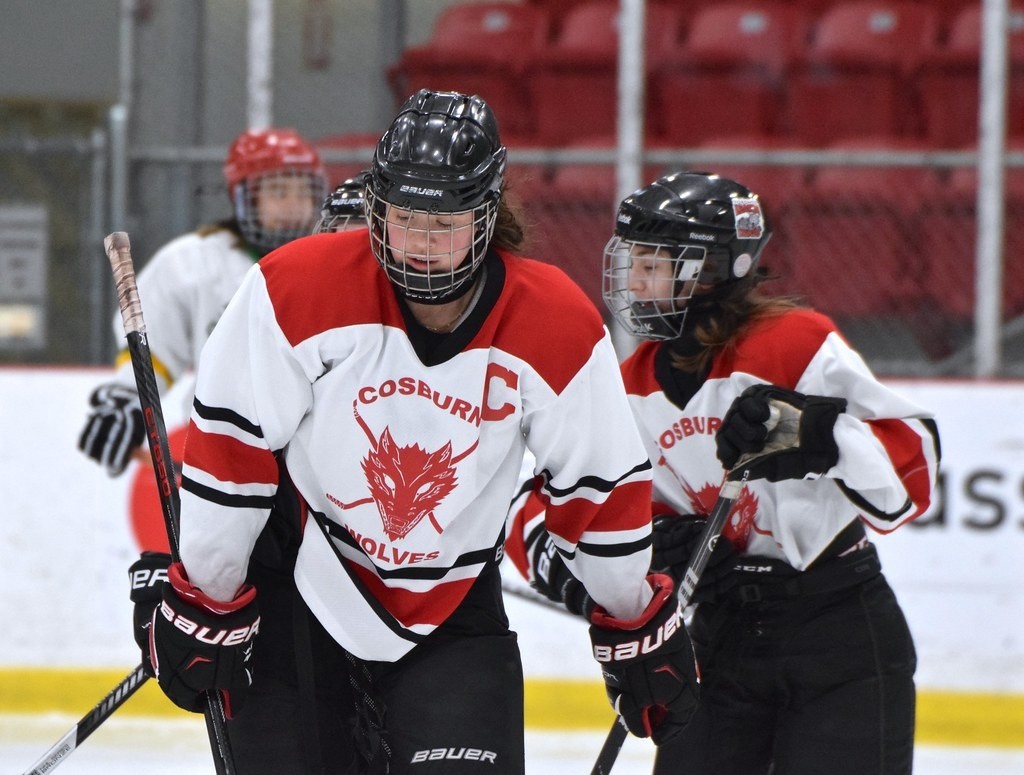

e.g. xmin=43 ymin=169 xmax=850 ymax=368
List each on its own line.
xmin=103 ymin=228 xmax=239 ymax=775
xmin=25 ymin=662 xmax=151 ymax=773
xmin=591 ymin=405 xmax=782 ymax=773
xmin=131 ymin=443 xmax=574 ymax=614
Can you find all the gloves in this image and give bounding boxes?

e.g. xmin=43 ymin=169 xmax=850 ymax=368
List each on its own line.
xmin=147 ymin=562 xmax=262 ymax=720
xmin=713 ymin=383 xmax=848 ymax=484
xmin=589 ymin=571 xmax=703 ymax=747
xmin=528 ymin=530 xmax=596 ymax=622
xmin=75 ymin=375 xmax=147 ymax=480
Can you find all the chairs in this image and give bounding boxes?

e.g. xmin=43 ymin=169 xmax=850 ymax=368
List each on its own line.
xmin=309 ymin=0 xmax=1024 ymax=363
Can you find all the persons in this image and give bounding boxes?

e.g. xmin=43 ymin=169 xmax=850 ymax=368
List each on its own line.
xmin=503 ymin=169 xmax=941 ymax=774
xmin=147 ymin=90 xmax=698 ymax=774
xmin=79 ymin=127 xmax=338 ymax=477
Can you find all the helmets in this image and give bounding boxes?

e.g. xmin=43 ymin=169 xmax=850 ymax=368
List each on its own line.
xmin=320 ymin=170 xmax=371 ymax=219
xmin=613 ymin=170 xmax=771 ymax=343
xmin=220 ymin=127 xmax=321 ymax=247
xmin=369 ymin=88 xmax=508 ymax=304
